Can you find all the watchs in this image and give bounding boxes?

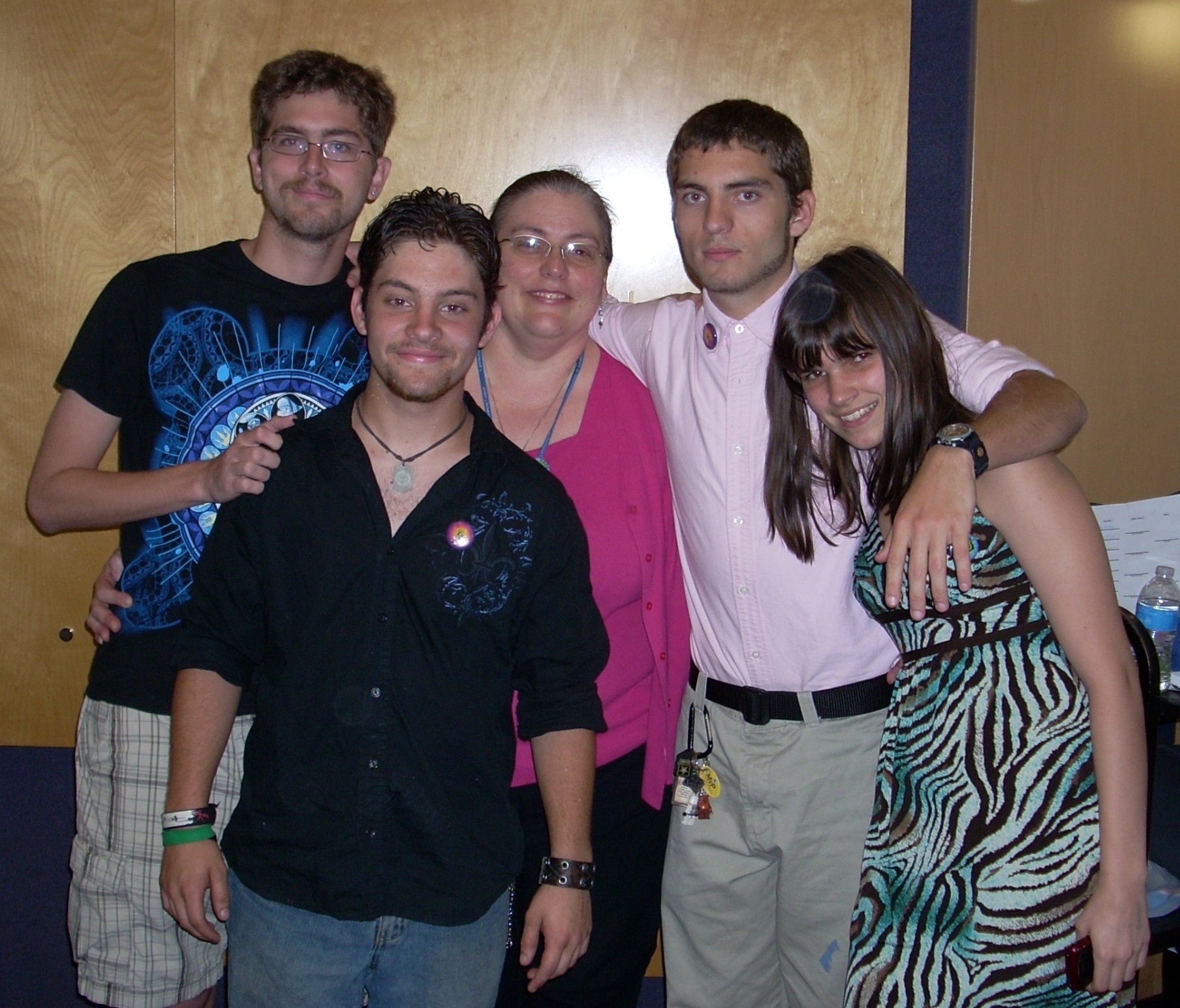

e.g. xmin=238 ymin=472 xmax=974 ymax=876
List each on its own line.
xmin=932 ymin=422 xmax=989 ymax=479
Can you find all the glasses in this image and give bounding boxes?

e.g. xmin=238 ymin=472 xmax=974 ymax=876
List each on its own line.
xmin=259 ymin=133 xmax=379 ymax=162
xmin=498 ymin=234 xmax=607 ymax=268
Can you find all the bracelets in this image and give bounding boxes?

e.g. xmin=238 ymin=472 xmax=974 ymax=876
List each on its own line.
xmin=538 ymin=854 xmax=599 ymax=892
xmin=159 ymin=804 xmax=220 ymax=844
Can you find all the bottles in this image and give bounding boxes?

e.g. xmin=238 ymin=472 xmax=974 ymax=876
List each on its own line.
xmin=1130 ymin=566 xmax=1179 ymax=692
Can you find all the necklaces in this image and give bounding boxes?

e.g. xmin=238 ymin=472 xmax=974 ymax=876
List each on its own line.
xmin=475 ymin=347 xmax=584 ymax=471
xmin=356 ymin=399 xmax=468 ymax=494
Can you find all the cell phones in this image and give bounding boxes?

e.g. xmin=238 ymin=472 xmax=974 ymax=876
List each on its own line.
xmin=1061 ymin=935 xmax=1095 ymax=992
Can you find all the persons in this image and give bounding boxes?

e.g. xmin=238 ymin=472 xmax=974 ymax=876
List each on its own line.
xmin=767 ymin=241 xmax=1151 ymax=1008
xmin=23 ymin=50 xmax=401 ymax=1008
xmin=346 ymin=96 xmax=1083 ymax=1008
xmin=82 ymin=170 xmax=691 ymax=1008
xmin=158 ymin=189 xmax=602 ymax=1008
